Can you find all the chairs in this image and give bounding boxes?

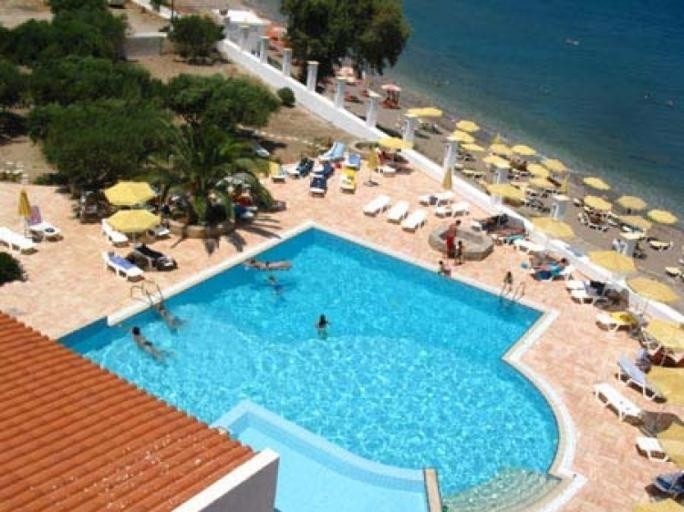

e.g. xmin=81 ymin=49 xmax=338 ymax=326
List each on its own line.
xmin=362 ymin=191 xmax=628 ymax=306
xmin=0 ymin=222 xmax=61 ymax=254
xmin=100 ymin=218 xmax=175 ymax=282
xmin=268 ymin=141 xmax=362 ymax=193
xmin=591 ymin=309 xmax=682 ymax=461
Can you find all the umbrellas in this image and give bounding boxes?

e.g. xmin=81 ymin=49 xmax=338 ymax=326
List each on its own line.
xmin=381 ymin=83 xmax=401 ymax=93
xmin=588 ymin=248 xmax=637 ymax=292
xmin=106 ymin=208 xmax=159 ymax=244
xmin=104 ymin=180 xmax=159 ymax=208
xmin=375 ymin=136 xmax=415 ymax=156
xmin=632 ymin=496 xmax=684 ymax=511
xmin=647 ymin=364 xmax=683 ymax=424
xmin=406 ymin=106 xmax=677 ymax=251
xmin=14 ymin=188 xmax=32 ymax=232
xmin=655 ymin=419 xmax=683 ymax=491
xmin=625 ymin=276 xmax=680 ymax=314
xmin=643 ymin=316 xmax=684 ymax=365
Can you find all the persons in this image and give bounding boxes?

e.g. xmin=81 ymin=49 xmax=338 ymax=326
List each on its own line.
xmin=316 ymin=313 xmax=331 ymax=337
xmin=435 ymin=260 xmax=452 ymax=276
xmin=263 ymin=275 xmax=289 ymax=303
xmin=452 ymin=239 xmax=464 ymax=265
xmin=532 ymin=257 xmax=570 ymax=273
xmin=443 ymin=219 xmax=461 ymax=256
xmin=500 ymin=262 xmax=512 ymax=291
xmin=242 ymin=257 xmax=291 ymax=270
xmin=131 ymin=326 xmax=173 ymax=365
xmin=155 ymin=299 xmax=185 ymax=333
xmin=235 ymin=188 xmax=253 ymax=207
xmin=375 ymin=146 xmax=390 ymax=167
